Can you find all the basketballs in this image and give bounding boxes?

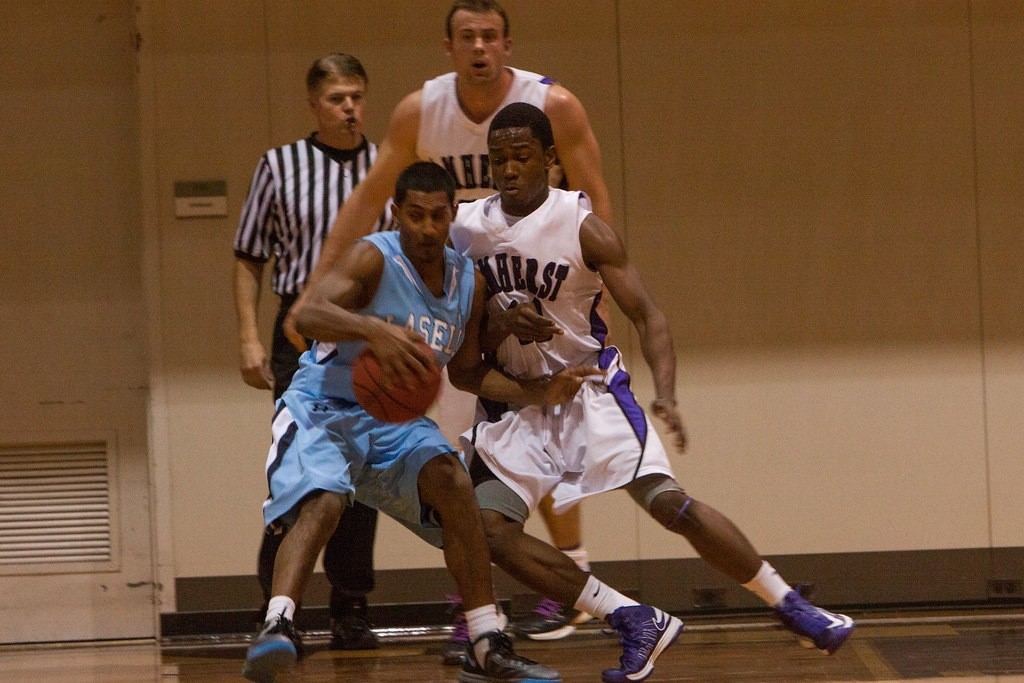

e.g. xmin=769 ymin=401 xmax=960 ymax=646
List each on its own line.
xmin=351 ymin=327 xmax=443 ymax=423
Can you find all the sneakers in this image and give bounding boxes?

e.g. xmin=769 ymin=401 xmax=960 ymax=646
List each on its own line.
xmin=457 ymin=628 xmax=563 ymax=683
xmin=775 ymin=584 xmax=856 ymax=657
xmin=601 ymin=603 xmax=684 ymax=683
xmin=515 ymin=594 xmax=595 ymax=641
xmin=252 ymin=613 xmax=305 ymax=661
xmin=329 ymin=600 xmax=381 ymax=650
xmin=241 ymin=606 xmax=297 ymax=683
xmin=445 ymin=592 xmax=469 ymax=666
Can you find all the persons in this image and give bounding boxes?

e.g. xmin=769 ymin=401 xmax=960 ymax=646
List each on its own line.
xmin=241 ymin=160 xmax=564 ymax=683
xmin=445 ymin=101 xmax=853 ymax=682
xmin=282 ymin=0 xmax=612 ymax=640
xmin=234 ymin=50 xmax=400 ymax=660
